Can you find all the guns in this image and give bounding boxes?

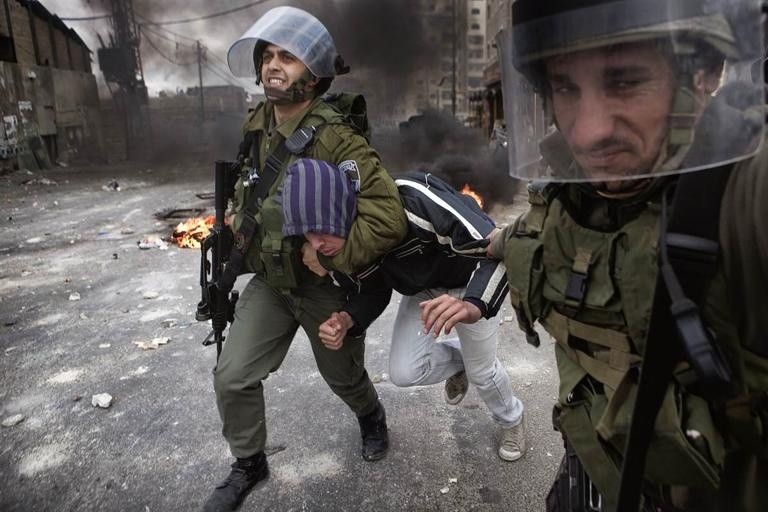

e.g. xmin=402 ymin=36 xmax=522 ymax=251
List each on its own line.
xmin=552 ymin=404 xmax=592 ymax=512
xmin=196 ymin=159 xmax=249 ymax=364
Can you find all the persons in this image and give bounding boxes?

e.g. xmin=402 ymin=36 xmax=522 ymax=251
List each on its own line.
xmin=281 ymin=158 xmax=525 ymax=462
xmin=483 ymin=0 xmax=767 ymax=512
xmin=201 ymin=4 xmax=408 ymax=512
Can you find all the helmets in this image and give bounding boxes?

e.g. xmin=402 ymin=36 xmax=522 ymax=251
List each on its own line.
xmin=495 ymin=0 xmax=768 ymax=182
xmin=228 ymin=6 xmax=345 ymax=103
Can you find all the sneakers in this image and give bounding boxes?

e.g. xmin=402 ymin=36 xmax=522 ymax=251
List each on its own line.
xmin=358 ymin=405 xmax=388 ymax=461
xmin=201 ymin=453 xmax=269 ymax=512
xmin=498 ymin=411 xmax=527 ymax=460
xmin=445 ymin=373 xmax=469 ymax=405
